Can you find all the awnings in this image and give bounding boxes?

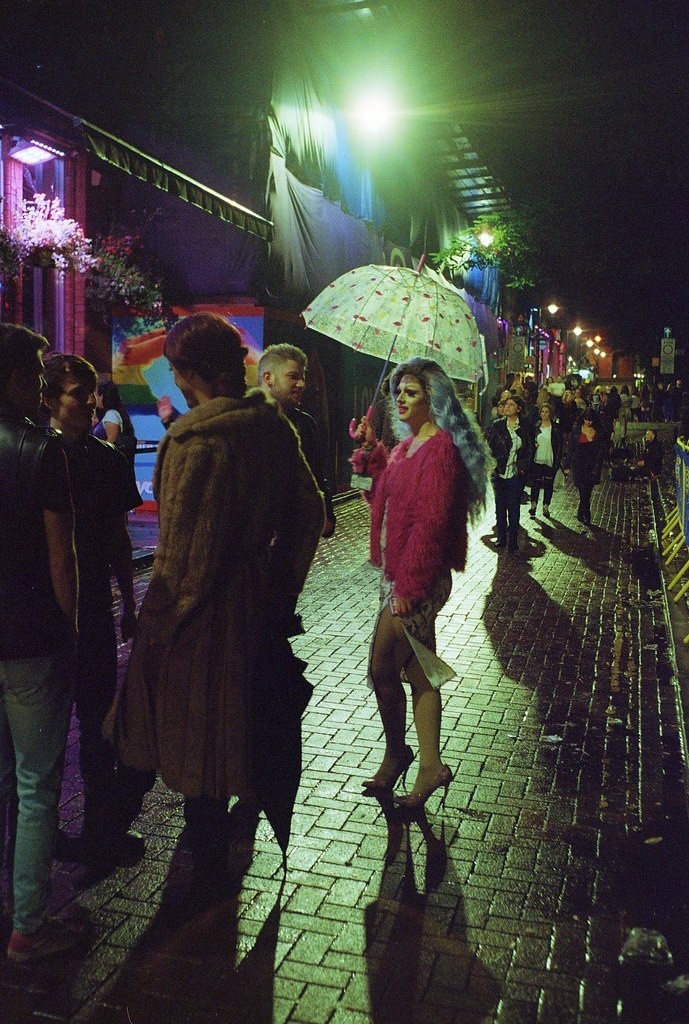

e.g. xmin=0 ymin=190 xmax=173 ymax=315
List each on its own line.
xmin=4 ymin=74 xmax=278 ymax=249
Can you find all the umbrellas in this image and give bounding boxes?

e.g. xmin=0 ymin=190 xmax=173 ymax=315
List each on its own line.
xmin=298 ymin=253 xmax=489 ymax=440
xmin=247 ymin=638 xmax=313 ymax=877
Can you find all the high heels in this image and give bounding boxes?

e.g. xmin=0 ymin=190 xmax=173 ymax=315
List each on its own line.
xmin=361 ymin=745 xmax=415 ymax=791
xmin=395 ymin=763 xmax=452 ymax=809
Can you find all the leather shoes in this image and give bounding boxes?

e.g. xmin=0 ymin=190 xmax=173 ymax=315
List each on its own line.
xmin=82 ymin=818 xmax=145 ymax=850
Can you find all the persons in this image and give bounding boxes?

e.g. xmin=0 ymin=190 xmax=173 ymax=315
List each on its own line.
xmin=113 ymin=310 xmax=324 ymax=838
xmin=258 ymin=343 xmax=336 ymax=537
xmin=43 ymin=354 xmax=147 ymax=849
xmin=93 ymin=380 xmax=181 ymax=626
xmin=486 ymin=395 xmax=535 ymax=551
xmin=348 ymin=357 xmax=498 ymax=808
xmin=0 ymin=322 xmax=79 ymax=962
xmin=372 ymin=372 xmax=684 ymax=484
xmin=529 ymin=403 xmax=562 ymax=517
xmin=563 ymin=408 xmax=605 ymax=526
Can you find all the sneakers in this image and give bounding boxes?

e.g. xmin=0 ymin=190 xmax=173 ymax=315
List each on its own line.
xmin=7 ymin=915 xmax=84 ymax=961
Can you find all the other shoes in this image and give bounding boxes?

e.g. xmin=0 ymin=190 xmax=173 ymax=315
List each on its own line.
xmin=508 ymin=538 xmax=519 ymax=550
xmin=494 ymin=537 xmax=506 ymax=547
xmin=543 ymin=511 xmax=550 ymax=518
xmin=529 ymin=509 xmax=536 ymax=516
xmin=577 ymin=510 xmax=591 ymax=525
